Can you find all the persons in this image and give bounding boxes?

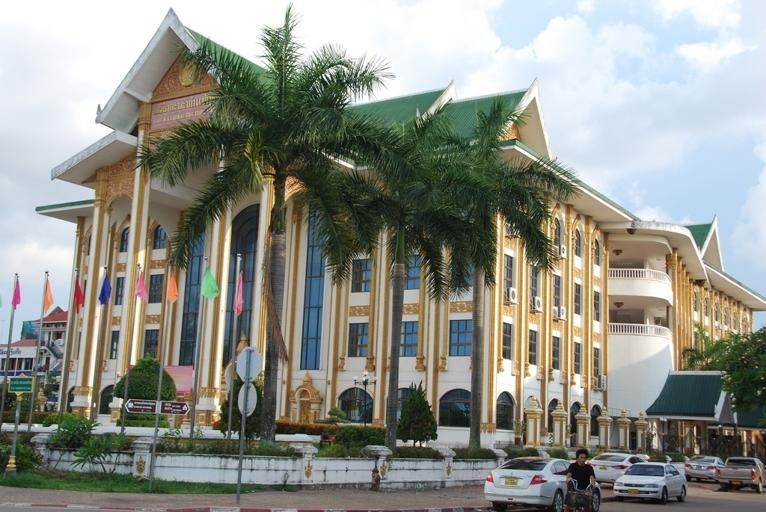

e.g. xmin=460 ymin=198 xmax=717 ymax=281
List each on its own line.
xmin=562 ymin=448 xmax=597 ymax=512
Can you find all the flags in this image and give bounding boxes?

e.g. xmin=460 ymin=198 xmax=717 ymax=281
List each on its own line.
xmin=199 ymin=267 xmax=219 ymax=300
xmin=43 ymin=280 xmax=55 ymax=315
xmin=73 ymin=275 xmax=83 ymax=314
xmin=97 ymin=275 xmax=112 ymax=305
xmin=165 ymin=268 xmax=179 ymax=305
xmin=233 ymin=269 xmax=245 ymax=317
xmin=134 ymin=270 xmax=149 ymax=303
xmin=10 ymin=279 xmax=20 ymax=308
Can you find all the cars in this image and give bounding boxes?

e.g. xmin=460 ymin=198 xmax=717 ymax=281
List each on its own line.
xmin=483 ymin=456 xmax=602 ymax=512
xmin=685 ymin=455 xmax=724 ymax=483
xmin=588 ymin=453 xmax=648 ymax=489
xmin=612 ymin=462 xmax=688 ymax=505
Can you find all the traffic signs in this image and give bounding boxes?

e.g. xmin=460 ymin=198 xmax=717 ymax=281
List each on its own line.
xmin=125 ymin=398 xmax=156 ymax=414
xmin=160 ymin=401 xmax=190 ymax=416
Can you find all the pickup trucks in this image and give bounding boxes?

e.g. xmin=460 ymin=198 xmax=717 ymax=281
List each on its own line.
xmin=716 ymin=457 xmax=766 ymax=493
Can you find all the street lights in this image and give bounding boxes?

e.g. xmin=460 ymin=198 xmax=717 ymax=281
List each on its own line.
xmin=353 ymin=371 xmax=376 ymax=428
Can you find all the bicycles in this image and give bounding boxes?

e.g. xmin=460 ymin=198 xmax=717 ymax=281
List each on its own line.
xmin=562 ymin=479 xmax=593 ymax=512
